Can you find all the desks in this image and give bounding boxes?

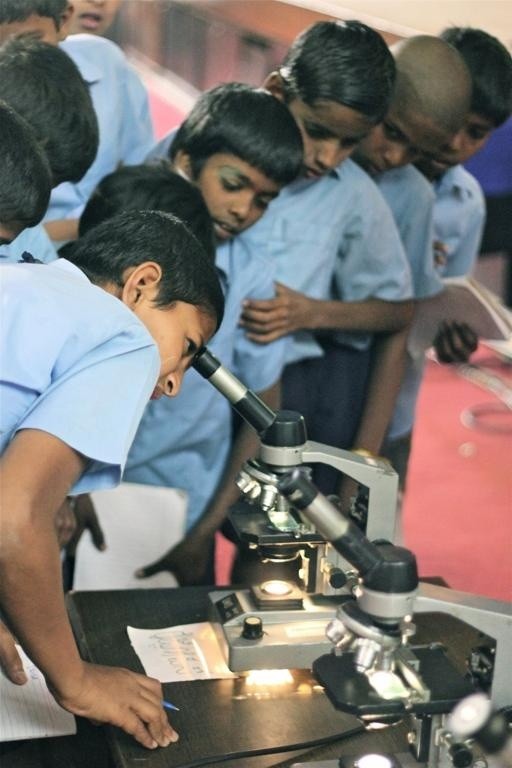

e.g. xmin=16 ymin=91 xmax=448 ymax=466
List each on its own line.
xmin=0 ymin=572 xmax=451 ymax=768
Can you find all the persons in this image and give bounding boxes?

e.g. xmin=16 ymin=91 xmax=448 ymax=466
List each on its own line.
xmin=1 ymin=205 xmax=229 ymax=753
xmin=2 ymin=2 xmax=510 ymax=589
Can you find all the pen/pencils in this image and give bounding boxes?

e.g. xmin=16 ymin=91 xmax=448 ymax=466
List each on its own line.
xmin=162 ymin=700 xmax=181 ymax=712
